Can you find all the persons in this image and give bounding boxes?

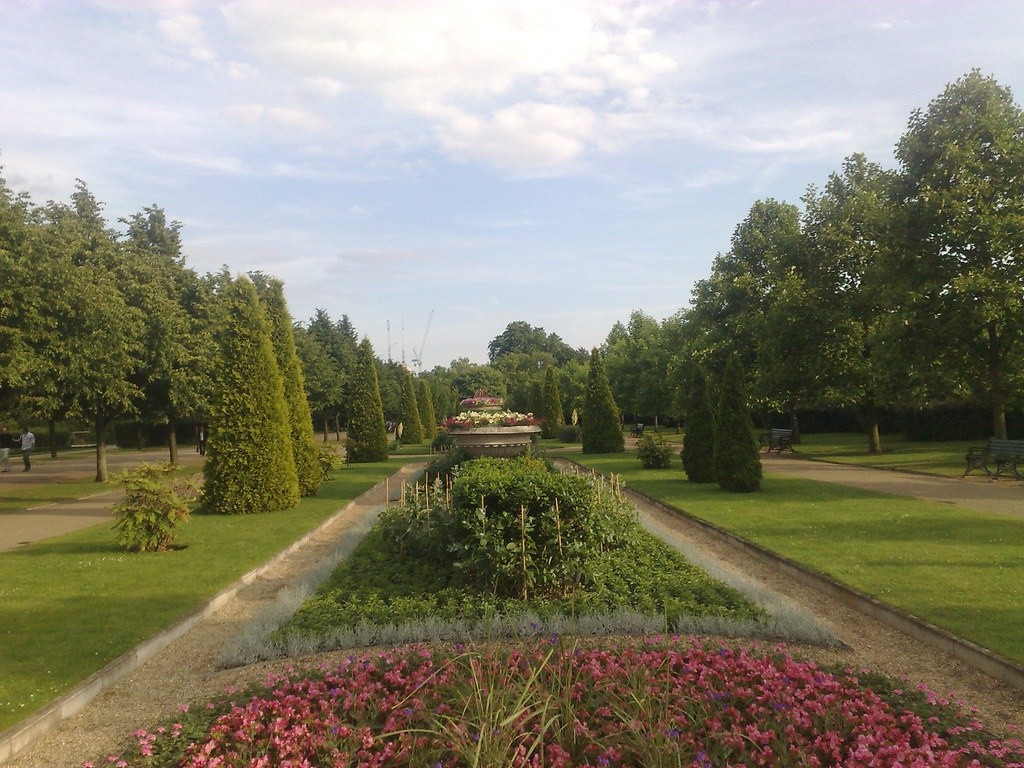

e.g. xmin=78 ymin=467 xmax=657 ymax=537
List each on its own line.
xmin=0 ymin=425 xmax=36 ymax=472
xmin=195 ymin=425 xmax=206 ymax=457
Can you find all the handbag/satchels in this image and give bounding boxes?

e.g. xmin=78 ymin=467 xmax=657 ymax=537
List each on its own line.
xmin=196 ymin=444 xmax=198 ymax=453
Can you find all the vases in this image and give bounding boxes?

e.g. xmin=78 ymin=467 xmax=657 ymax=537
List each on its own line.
xmin=461 ymin=405 xmax=502 ymax=413
xmin=447 ymin=425 xmax=542 ymax=459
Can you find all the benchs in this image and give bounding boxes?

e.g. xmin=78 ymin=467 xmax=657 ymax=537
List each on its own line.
xmin=759 ymin=428 xmax=795 ymax=455
xmin=631 ymin=423 xmax=644 ymax=437
xmin=962 ymin=439 xmax=1023 ymax=481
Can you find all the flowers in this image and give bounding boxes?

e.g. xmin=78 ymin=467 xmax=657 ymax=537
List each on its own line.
xmin=442 ymin=397 xmax=538 ymax=428
